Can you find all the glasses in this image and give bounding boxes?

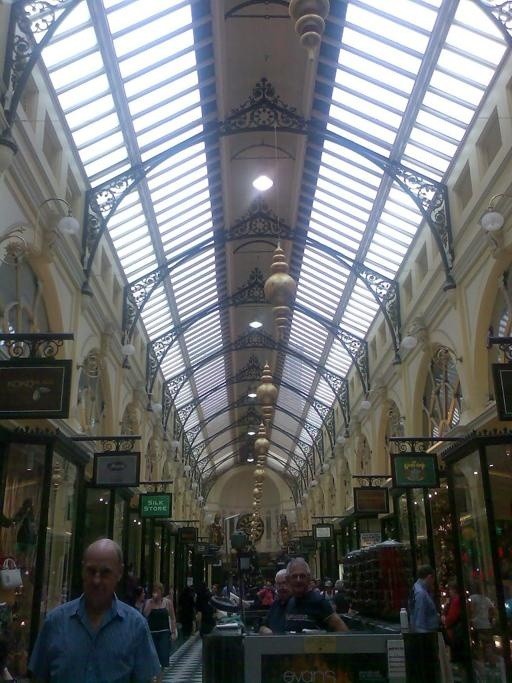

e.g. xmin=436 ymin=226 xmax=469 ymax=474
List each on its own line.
xmin=83 ymin=566 xmax=112 ymax=579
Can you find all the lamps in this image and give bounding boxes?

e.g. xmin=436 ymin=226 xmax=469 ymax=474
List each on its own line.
xmin=0 ymin=199 xmax=80 ymax=267
xmin=77 ymin=332 xmax=136 ymax=377
xmin=480 ymin=194 xmax=512 ymax=232
xmin=294 ymin=320 xmax=424 ymax=511
xmin=120 ymin=384 xmax=213 ymax=505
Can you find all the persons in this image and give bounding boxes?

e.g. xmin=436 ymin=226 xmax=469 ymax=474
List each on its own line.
xmin=124 ymin=557 xmax=501 ymax=682
xmin=25 ymin=537 xmax=164 ymax=682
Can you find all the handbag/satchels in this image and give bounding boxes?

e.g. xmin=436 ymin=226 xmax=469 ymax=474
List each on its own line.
xmin=1 ymin=567 xmax=22 ymax=590
xmin=168 ymin=616 xmax=178 ymax=641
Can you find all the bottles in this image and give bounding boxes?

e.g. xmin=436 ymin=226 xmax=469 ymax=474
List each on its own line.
xmin=399 ymin=607 xmax=408 ymax=628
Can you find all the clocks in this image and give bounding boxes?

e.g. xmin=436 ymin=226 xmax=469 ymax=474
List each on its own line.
xmin=236 ymin=513 xmax=264 ymax=542
